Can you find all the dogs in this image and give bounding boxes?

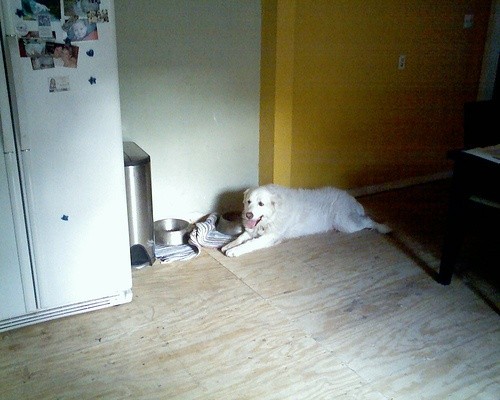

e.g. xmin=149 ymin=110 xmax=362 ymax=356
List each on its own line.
xmin=220 ymin=183 xmax=393 ymax=259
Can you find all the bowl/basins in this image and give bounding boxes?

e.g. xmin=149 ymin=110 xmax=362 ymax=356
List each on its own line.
xmin=216 ymin=209 xmax=246 ymax=236
xmin=153 ymin=219 xmax=187 ymax=247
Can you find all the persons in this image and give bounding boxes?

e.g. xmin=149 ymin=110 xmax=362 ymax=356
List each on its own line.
xmin=48 ymin=78 xmax=56 ymax=92
xmin=71 ymin=21 xmax=91 ymax=42
xmin=51 ymin=46 xmax=64 ymax=65
xmin=61 ymin=47 xmax=76 ymax=67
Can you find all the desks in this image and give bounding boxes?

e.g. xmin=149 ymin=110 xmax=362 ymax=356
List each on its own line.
xmin=438 ymin=147 xmax=500 ymax=285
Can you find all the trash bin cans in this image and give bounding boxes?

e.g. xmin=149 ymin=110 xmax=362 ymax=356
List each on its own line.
xmin=123 ymin=142 xmax=156 ymax=269
